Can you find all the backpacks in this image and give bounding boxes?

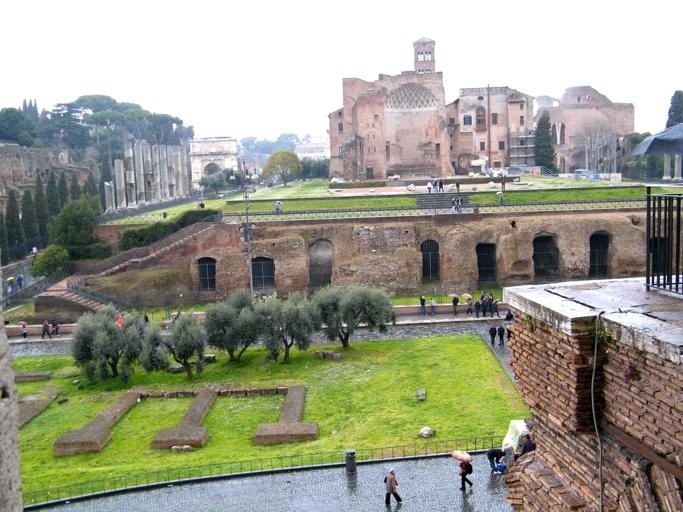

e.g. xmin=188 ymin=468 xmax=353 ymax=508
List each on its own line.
xmin=464 ymin=462 xmax=473 ymax=475
xmin=497 ymin=463 xmax=506 ymax=473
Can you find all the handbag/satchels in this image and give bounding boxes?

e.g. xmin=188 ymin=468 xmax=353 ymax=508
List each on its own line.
xmin=384 ymin=476 xmax=387 ymax=483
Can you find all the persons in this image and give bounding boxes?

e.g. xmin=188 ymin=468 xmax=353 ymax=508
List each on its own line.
xmin=499 ymin=172 xmax=506 ymax=191
xmin=458 ymin=460 xmax=472 ymax=491
xmin=486 ymin=449 xmax=505 ymax=474
xmin=419 ymin=290 xmax=513 ymax=346
xmin=40 ymin=319 xmax=54 ymax=339
xmin=7 ymin=279 xmax=14 ymax=293
xmin=174 ymin=310 xmax=182 ymax=320
xmin=383 ymin=469 xmax=401 ymax=505
xmin=498 ymin=194 xmax=504 ymax=208
xmin=272 ymin=203 xmax=282 ymax=219
xmin=16 ymin=276 xmax=22 ymax=288
xmin=517 ymin=434 xmax=534 ymax=458
xmin=50 ymin=320 xmax=60 ymax=338
xmin=426 ymin=178 xmax=462 ymax=212
xmin=143 ymin=313 xmax=148 ymax=325
xmin=21 ymin=324 xmax=27 ymax=339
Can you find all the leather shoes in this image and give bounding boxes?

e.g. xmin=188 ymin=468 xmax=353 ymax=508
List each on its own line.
xmin=385 ymin=499 xmax=403 ymax=505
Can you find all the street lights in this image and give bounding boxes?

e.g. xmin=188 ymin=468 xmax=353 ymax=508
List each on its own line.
xmin=230 ymin=161 xmax=259 ymax=304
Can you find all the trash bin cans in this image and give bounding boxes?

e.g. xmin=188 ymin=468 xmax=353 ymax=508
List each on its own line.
xmin=345 ymin=449 xmax=358 ymax=474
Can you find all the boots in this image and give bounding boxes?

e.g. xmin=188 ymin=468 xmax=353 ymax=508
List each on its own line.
xmin=459 ymin=481 xmax=474 ymax=490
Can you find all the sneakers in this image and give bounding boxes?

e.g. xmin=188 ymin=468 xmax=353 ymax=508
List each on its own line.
xmin=491 ymin=467 xmax=501 ymax=474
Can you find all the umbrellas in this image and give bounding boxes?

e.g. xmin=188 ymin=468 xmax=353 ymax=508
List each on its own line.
xmin=450 ymin=451 xmax=472 ymax=464
xmin=495 ymin=191 xmax=502 ymax=194
xmin=5 ymin=277 xmax=14 ymax=282
xmin=17 ymin=320 xmax=28 ymax=325
xmin=275 ymin=200 xmax=282 ymax=204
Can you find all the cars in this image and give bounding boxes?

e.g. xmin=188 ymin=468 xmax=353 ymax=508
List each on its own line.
xmin=216 ymin=194 xmax=224 ymax=198
